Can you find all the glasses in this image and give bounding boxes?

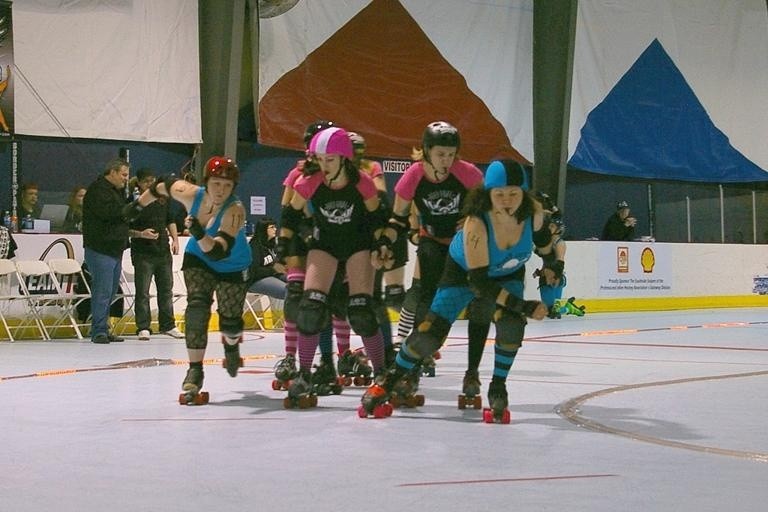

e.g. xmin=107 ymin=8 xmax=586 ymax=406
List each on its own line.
xmin=267 ymin=226 xmax=275 ymax=230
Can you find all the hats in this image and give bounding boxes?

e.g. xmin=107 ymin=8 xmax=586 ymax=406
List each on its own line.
xmin=136 ymin=167 xmax=161 ymax=182
xmin=617 ymin=200 xmax=632 ymax=210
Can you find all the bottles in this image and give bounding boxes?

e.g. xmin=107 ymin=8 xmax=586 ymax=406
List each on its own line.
xmin=3 ymin=211 xmax=11 ymax=227
xmin=10 ymin=209 xmax=18 ymax=233
xmin=133 ymin=187 xmax=141 ymax=200
xmin=23 ymin=214 xmax=33 ymax=229
xmin=244 ymin=221 xmax=249 ymax=236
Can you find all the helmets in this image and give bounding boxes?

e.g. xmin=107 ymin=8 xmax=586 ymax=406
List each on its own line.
xmin=309 ymin=127 xmax=353 ymax=160
xmin=550 ymin=218 xmax=565 ymax=235
xmin=422 ymin=121 xmax=459 ymax=154
xmin=303 ymin=120 xmax=336 ymax=150
xmin=484 ymin=158 xmax=528 ymax=191
xmin=347 ymin=132 xmax=366 ymax=153
xmin=203 ymin=156 xmax=239 ymax=184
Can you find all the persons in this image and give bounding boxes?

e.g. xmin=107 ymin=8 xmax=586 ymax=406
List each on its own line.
xmin=601 ymin=199 xmax=638 ymax=240
xmin=125 ymin=166 xmax=187 ymax=341
xmin=274 ymin=119 xmax=393 ymax=414
xmin=65 ymin=187 xmax=88 ymax=232
xmin=247 ymin=216 xmax=289 ymax=302
xmin=529 ymin=189 xmax=559 ymax=222
xmin=360 ymin=159 xmax=556 ymax=410
xmin=378 ymin=120 xmax=490 ymax=398
xmin=82 ymin=157 xmax=131 ymax=344
xmin=123 ymin=155 xmax=253 ymax=393
xmin=395 ymin=253 xmax=421 ymax=341
xmin=536 ymin=215 xmax=584 ymax=319
xmin=16 ymin=181 xmax=39 ymax=227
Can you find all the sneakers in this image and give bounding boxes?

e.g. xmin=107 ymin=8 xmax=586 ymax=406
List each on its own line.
xmin=138 ymin=330 xmax=151 ymax=340
xmin=164 ymin=327 xmax=185 ymax=338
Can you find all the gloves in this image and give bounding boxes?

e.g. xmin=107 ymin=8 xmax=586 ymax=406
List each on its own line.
xmin=121 ymin=199 xmax=144 ymax=224
xmin=546 ymin=259 xmax=563 ymax=280
xmin=370 ymin=235 xmax=392 ymax=256
xmin=273 ymin=236 xmax=291 ymax=265
xmin=505 ymin=293 xmax=540 ymax=318
xmin=188 ymin=214 xmax=205 ymax=241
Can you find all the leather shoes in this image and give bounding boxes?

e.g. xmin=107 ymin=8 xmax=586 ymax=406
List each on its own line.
xmin=108 ymin=335 xmax=124 ymax=342
xmin=91 ymin=335 xmax=109 ymax=344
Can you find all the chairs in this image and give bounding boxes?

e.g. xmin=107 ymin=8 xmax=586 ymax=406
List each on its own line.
xmin=0 ymin=257 xmax=285 ymax=342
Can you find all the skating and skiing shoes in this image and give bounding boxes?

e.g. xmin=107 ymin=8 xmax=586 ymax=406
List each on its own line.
xmin=179 ymin=367 xmax=208 ymax=405
xmin=484 ymin=381 xmax=510 ymax=424
xmin=221 ymin=335 xmax=244 ymax=378
xmin=457 ymin=371 xmax=481 ymax=409
xmin=313 ymin=358 xmax=343 ymax=396
xmin=391 ymin=370 xmax=424 ymax=408
xmin=358 ymin=363 xmax=409 ymax=419
xmin=338 ymin=349 xmax=372 ymax=386
xmin=272 ymin=354 xmax=298 ymax=390
xmin=284 ymin=370 xmax=317 ymax=409
